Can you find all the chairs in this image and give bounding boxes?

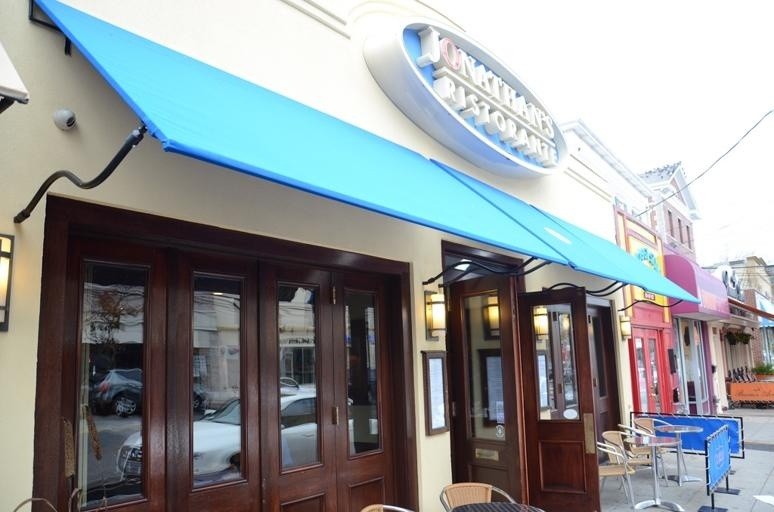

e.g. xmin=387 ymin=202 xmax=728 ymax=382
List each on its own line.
xmin=595 ymin=415 xmax=707 ymax=512
xmin=441 ymin=476 xmax=514 ymax=509
xmin=723 ymin=364 xmax=758 ymax=383
xmin=361 ymin=499 xmax=409 ymax=512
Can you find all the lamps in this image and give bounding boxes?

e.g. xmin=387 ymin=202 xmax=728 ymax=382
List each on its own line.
xmin=619 ymin=313 xmax=633 ymax=341
xmin=482 ymin=295 xmax=501 ymax=341
xmin=425 ymin=287 xmax=449 ymax=343
xmin=0 ymin=231 xmax=15 ymax=331
xmin=533 ymin=305 xmax=550 ymax=343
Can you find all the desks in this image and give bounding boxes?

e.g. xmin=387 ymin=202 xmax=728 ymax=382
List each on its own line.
xmin=451 ymin=501 xmax=544 ymax=512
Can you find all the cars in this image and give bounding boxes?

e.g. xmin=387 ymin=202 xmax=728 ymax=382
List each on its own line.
xmin=92 ymin=367 xmax=206 ymax=418
xmin=342 ymin=366 xmax=377 ymax=417
xmin=543 ymin=359 xmax=574 ymax=388
xmin=113 ymin=375 xmax=377 ymax=491
xmin=636 ymin=359 xmax=658 ymax=393
